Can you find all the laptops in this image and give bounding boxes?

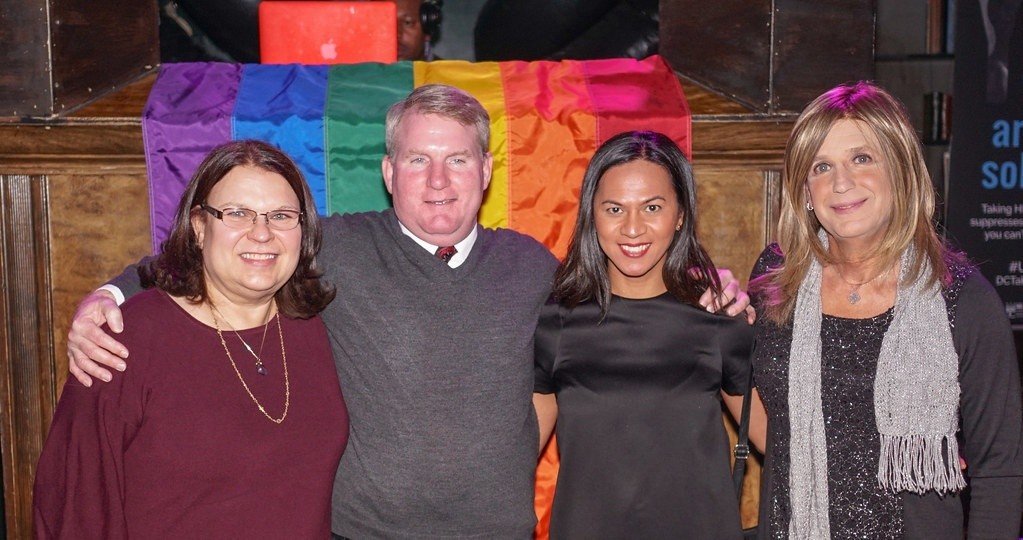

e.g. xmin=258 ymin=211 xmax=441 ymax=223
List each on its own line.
xmin=259 ymin=1 xmax=398 ymax=65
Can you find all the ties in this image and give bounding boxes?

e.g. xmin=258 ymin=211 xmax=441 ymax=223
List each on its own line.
xmin=435 ymin=246 xmax=458 ymax=264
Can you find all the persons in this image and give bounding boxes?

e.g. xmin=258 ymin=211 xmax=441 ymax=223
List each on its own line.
xmin=67 ymin=82 xmax=760 ymax=540
xmin=536 ymin=130 xmax=755 ymax=539
xmin=741 ymin=83 xmax=1023 ymax=540
xmin=29 ymin=140 xmax=347 ymax=540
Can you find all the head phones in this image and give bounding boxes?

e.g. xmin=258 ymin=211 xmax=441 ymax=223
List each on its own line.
xmin=419 ymin=0 xmax=442 ymax=41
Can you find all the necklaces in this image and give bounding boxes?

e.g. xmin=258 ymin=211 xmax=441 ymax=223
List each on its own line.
xmin=826 ymin=249 xmax=898 ymax=303
xmin=201 ymin=293 xmax=294 ymax=424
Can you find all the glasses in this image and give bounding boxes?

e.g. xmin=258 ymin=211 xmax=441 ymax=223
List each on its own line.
xmin=202 ymin=202 xmax=304 ymax=232
